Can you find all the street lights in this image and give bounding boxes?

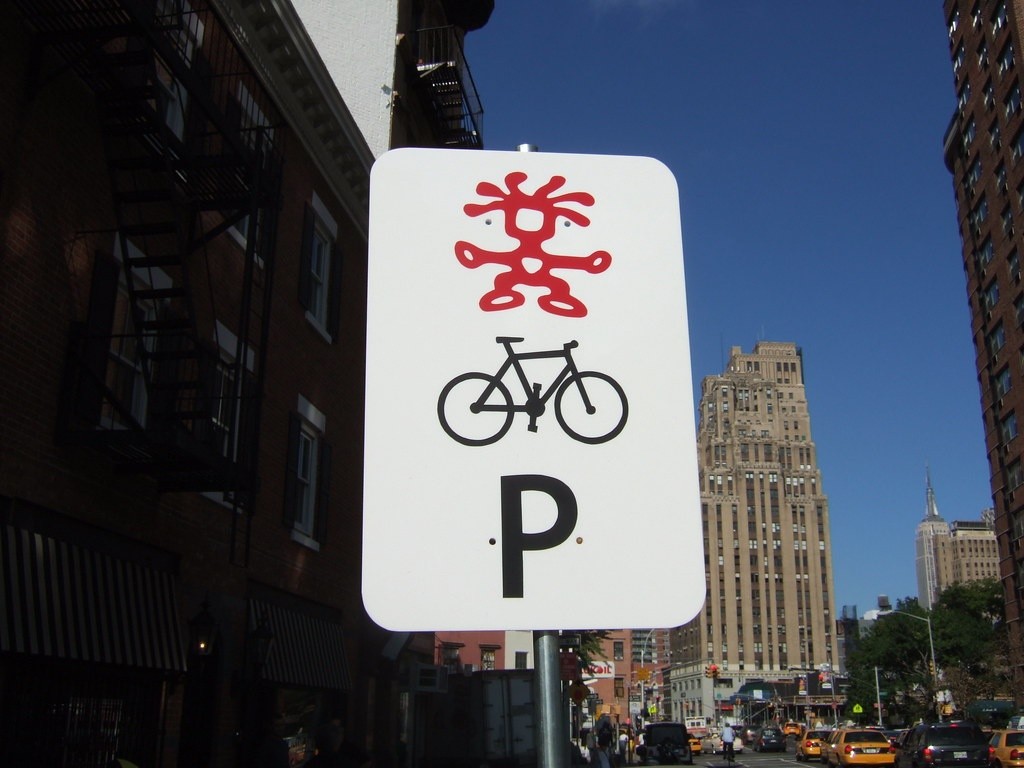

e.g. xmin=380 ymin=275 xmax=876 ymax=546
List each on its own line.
xmin=875 ymin=610 xmax=938 ymax=683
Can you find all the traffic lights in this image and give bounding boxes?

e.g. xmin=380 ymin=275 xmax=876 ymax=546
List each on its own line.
xmin=705 ymin=665 xmax=721 ymax=680
xmin=928 ymin=659 xmax=934 ymax=676
xmin=656 ymin=695 xmax=661 ymax=702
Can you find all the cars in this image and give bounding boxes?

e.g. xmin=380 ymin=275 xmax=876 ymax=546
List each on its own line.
xmin=751 ymin=727 xmax=789 ymax=752
xmin=794 ymin=729 xmax=831 ymax=761
xmin=784 ymin=721 xmax=801 ymax=736
xmin=638 ymin=722 xmax=701 ymax=765
xmin=815 ymin=721 xmax=1024 ymax=767
xmin=701 ymin=724 xmax=760 ymax=756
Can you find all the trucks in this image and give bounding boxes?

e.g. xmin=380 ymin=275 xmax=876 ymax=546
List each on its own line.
xmin=685 ymin=717 xmax=710 ymax=729
xmin=721 ymin=716 xmax=745 ymax=736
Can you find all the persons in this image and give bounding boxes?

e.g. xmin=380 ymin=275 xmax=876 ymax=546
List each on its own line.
xmin=619 ymin=733 xmax=629 ymax=755
xmin=719 ymin=722 xmax=735 ymax=762
xmin=597 ymin=722 xmax=613 ymax=759
xmin=628 ymin=727 xmax=635 ymax=762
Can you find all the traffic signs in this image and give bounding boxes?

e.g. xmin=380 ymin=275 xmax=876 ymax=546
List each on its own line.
xmin=585 ymin=693 xmax=603 ymax=705
xmin=557 ymin=634 xmax=581 ymax=649
